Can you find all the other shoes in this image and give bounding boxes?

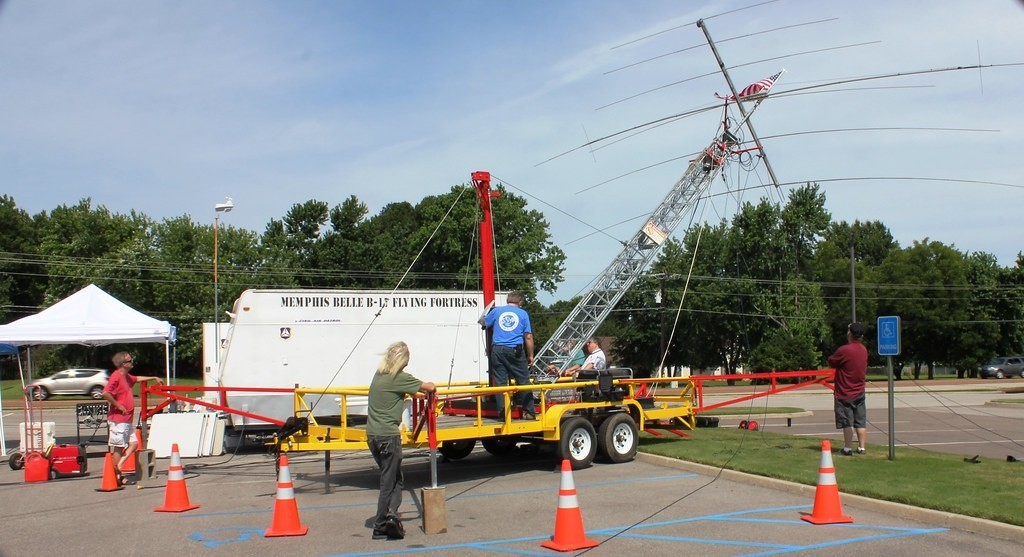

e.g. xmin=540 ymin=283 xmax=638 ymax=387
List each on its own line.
xmin=522 ymin=412 xmax=535 ymax=420
xmin=835 ymin=448 xmax=853 ymax=456
xmin=372 ymin=529 xmax=389 ymax=539
xmin=385 ymin=517 xmax=405 ymax=540
xmin=856 ymin=448 xmax=866 ymax=455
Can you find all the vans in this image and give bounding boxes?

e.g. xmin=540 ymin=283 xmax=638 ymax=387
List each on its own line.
xmin=981 ymin=357 xmax=1024 ymax=379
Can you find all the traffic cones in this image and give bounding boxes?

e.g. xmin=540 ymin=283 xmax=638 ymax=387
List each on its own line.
xmin=264 ymin=455 xmax=308 ymax=537
xmin=153 ymin=443 xmax=200 ymax=512
xmin=540 ymin=459 xmax=600 ymax=552
xmin=95 ymin=453 xmax=125 ymax=492
xmin=800 ymin=439 xmax=853 ymax=525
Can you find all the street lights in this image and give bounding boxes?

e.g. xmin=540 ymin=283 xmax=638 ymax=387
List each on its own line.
xmin=216 ymin=203 xmax=233 ymax=364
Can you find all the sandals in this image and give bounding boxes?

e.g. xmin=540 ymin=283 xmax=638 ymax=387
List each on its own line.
xmin=122 ymin=475 xmax=137 ymax=484
xmin=115 ymin=465 xmax=122 ymax=488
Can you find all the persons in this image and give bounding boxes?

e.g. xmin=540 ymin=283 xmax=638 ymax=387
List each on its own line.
xmin=546 ymin=338 xmax=585 ymax=377
xmin=364 ymin=342 xmax=436 ymax=541
xmin=103 ymin=351 xmax=165 ymax=485
xmin=564 ymin=337 xmax=607 ymax=380
xmin=478 ymin=291 xmax=536 ymax=422
xmin=828 ymin=323 xmax=868 ymax=456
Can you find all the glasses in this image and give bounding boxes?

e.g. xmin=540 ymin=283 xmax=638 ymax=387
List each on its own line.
xmin=588 ymin=341 xmax=596 ymax=344
xmin=123 ymin=359 xmax=134 ymax=364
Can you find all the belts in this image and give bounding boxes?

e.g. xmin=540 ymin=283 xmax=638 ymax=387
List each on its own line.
xmin=493 ymin=344 xmax=522 ymax=350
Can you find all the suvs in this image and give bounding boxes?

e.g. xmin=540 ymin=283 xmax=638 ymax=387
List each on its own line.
xmin=30 ymin=368 xmax=108 ymax=400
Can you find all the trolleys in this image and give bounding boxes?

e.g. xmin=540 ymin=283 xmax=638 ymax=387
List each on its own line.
xmin=9 ymin=384 xmax=44 ymax=470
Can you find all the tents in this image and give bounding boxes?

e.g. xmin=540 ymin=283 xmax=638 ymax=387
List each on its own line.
xmin=0 ymin=283 xmax=177 ymax=457
xmin=1 ymin=343 xmax=30 ymax=455
